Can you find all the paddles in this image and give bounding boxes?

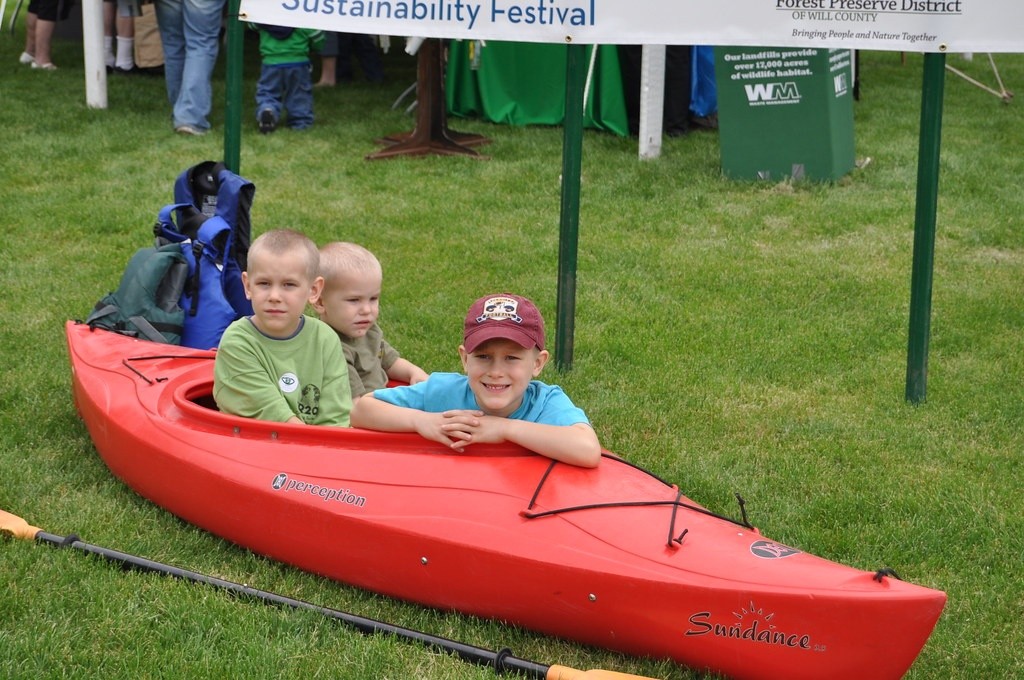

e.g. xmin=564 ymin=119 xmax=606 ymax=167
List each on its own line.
xmin=0 ymin=510 xmax=657 ymax=680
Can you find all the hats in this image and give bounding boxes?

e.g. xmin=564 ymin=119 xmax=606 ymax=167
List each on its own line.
xmin=463 ymin=292 xmax=545 ymax=354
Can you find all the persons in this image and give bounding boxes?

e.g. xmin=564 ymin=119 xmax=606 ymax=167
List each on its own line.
xmin=313 ymin=243 xmax=429 ymax=405
xmin=19 ymin=0 xmax=338 ymax=138
xmin=349 ymin=292 xmax=601 ymax=468
xmin=213 ymin=231 xmax=353 ymax=428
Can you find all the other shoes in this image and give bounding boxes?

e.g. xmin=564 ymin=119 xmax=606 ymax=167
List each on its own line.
xmin=105 ymin=65 xmax=114 ymax=76
xmin=19 ymin=52 xmax=34 ymax=63
xmin=177 ymin=124 xmax=206 ymax=136
xmin=30 ymin=61 xmax=57 ymax=71
xmin=114 ymin=66 xmax=136 ymax=75
xmin=259 ymin=109 xmax=275 ymax=134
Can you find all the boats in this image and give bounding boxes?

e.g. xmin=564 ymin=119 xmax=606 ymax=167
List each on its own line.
xmin=63 ymin=320 xmax=949 ymax=680
